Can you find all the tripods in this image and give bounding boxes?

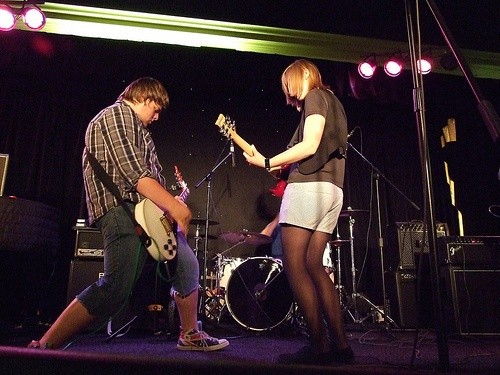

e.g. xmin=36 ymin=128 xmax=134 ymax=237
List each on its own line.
xmin=336 ymin=213 xmax=400 ymax=332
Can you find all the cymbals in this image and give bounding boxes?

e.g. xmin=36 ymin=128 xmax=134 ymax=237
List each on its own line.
xmin=189 ymin=218 xmax=219 ymax=226
xmin=340 ymin=210 xmax=369 ymax=216
xmin=329 ymin=239 xmax=350 ymax=246
xmin=216 ymin=231 xmax=273 ymax=246
xmin=190 ymin=233 xmax=218 ymax=240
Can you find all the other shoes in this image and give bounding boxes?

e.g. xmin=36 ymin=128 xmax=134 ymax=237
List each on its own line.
xmin=308 ymin=347 xmax=355 ymax=363
xmin=278 ymin=346 xmax=322 ymax=364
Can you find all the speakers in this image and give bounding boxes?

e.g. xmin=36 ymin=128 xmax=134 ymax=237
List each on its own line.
xmin=65 ymin=259 xmax=128 ymax=332
xmin=395 ymin=221 xmax=448 ymax=270
xmin=393 ymin=268 xmax=438 ymax=330
xmin=439 ymin=264 xmax=500 ymax=337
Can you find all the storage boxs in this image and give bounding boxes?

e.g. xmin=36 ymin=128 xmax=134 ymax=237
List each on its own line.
xmin=391 ymin=271 xmax=418 ymax=331
xmin=74 ymin=230 xmax=104 ymax=259
xmin=441 ymin=266 xmax=500 ymax=336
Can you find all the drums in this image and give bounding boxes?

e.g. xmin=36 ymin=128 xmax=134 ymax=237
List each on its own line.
xmin=225 ymin=256 xmax=294 ymax=331
xmin=219 ymin=258 xmax=245 ymax=288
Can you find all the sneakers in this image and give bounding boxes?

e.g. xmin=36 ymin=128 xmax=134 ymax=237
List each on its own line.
xmin=176 ymin=326 xmax=229 ymax=352
xmin=27 ymin=340 xmax=40 ymax=349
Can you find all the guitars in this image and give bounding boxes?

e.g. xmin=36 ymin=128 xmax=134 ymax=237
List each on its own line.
xmin=135 ymin=167 xmax=190 ymax=264
xmin=215 ymin=113 xmax=291 ymax=199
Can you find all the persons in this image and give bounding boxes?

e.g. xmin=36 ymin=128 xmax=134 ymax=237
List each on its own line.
xmin=28 ymin=77 xmax=229 ymax=351
xmin=259 ymin=179 xmax=335 ymax=321
xmin=242 ymin=59 xmax=356 ymax=365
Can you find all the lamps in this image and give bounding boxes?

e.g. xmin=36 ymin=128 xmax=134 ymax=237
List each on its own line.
xmin=439 ymin=50 xmax=463 ymax=71
xmin=20 ymin=4 xmax=46 ymax=31
xmin=416 ymin=52 xmax=435 ymax=75
xmin=383 ymin=53 xmax=406 ymax=78
xmin=358 ymin=54 xmax=380 ymax=80
xmin=0 ymin=4 xmax=17 ymax=31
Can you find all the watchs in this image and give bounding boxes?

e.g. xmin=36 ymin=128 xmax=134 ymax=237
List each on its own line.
xmin=265 ymin=158 xmax=270 ymax=170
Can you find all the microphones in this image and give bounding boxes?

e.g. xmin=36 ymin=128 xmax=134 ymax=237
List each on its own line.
xmin=230 ymin=140 xmax=236 ymax=167
